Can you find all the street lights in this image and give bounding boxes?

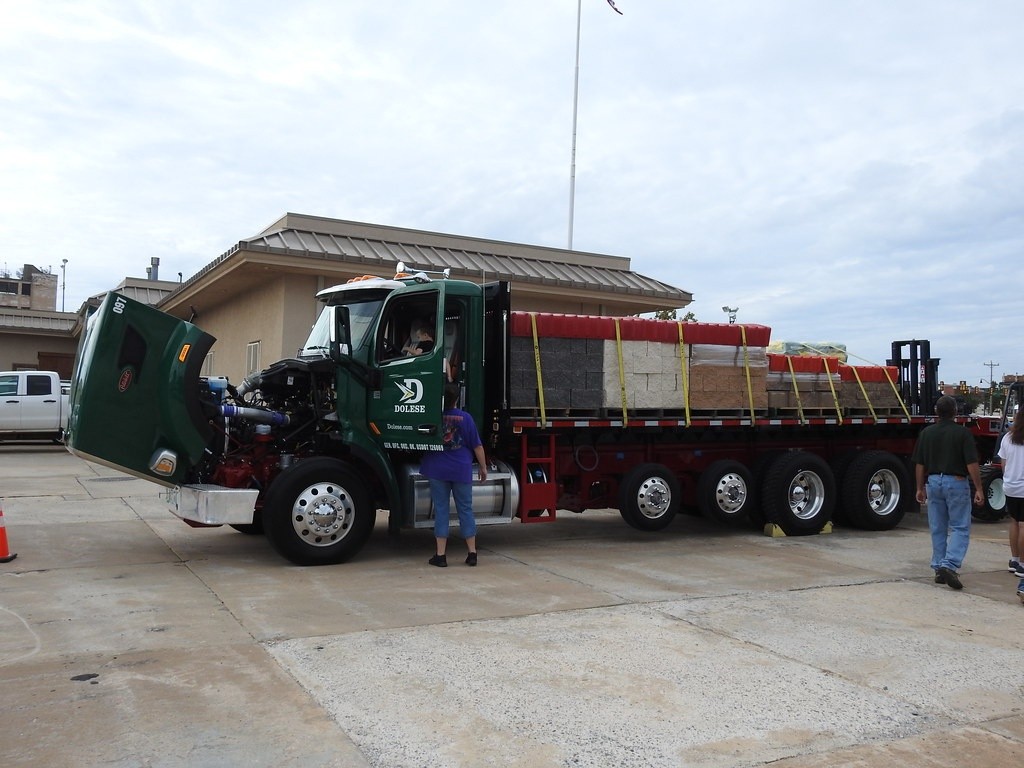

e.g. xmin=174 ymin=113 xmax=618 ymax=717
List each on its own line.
xmin=723 ymin=305 xmax=738 ymax=323
xmin=979 ymin=379 xmax=992 ymax=416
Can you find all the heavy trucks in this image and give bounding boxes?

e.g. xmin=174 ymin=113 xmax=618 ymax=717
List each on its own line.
xmin=62 ymin=265 xmax=1010 ymax=567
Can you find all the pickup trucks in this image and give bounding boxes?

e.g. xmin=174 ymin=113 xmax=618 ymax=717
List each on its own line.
xmin=0 ymin=371 xmax=69 ymax=441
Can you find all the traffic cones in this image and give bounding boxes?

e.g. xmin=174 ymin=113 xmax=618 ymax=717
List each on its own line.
xmin=1 ymin=509 xmax=19 ymax=562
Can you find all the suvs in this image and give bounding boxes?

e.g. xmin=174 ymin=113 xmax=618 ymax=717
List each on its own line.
xmin=0 ymin=380 xmax=70 ymax=395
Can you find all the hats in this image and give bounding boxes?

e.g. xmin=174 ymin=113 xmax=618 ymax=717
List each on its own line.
xmin=443 ymin=383 xmax=459 ymax=410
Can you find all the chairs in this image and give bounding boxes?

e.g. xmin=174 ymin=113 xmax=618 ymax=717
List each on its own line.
xmin=401 ymin=319 xmax=459 ymax=362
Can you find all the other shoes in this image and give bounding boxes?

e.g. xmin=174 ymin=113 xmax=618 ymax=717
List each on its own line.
xmin=934 ymin=571 xmax=946 ymax=584
xmin=1016 ymin=579 xmax=1024 ymax=598
xmin=428 ymin=552 xmax=447 ymax=567
xmin=939 ymin=567 xmax=963 ymax=590
xmin=1007 ymin=559 xmax=1019 ymax=572
xmin=465 ymin=551 xmax=477 ymax=566
xmin=1014 ymin=566 xmax=1024 ymax=578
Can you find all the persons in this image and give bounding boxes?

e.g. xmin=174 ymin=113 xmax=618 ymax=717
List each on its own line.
xmin=910 ymin=395 xmax=985 ymax=590
xmin=419 ymin=383 xmax=488 ymax=568
xmin=997 ymin=404 xmax=1024 ymax=607
xmin=401 ymin=324 xmax=453 ymax=383
